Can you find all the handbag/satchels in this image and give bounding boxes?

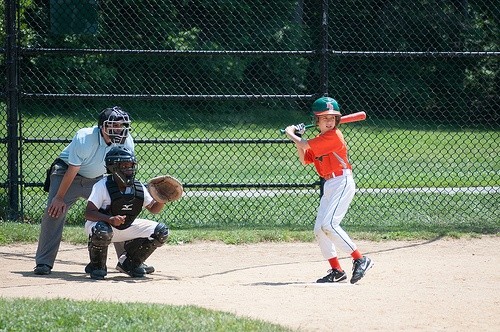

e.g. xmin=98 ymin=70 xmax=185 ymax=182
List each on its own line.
xmin=45 ymin=164 xmax=53 ymax=192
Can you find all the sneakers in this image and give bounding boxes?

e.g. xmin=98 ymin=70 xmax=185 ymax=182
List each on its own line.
xmin=350 ymin=257 xmax=373 ymax=284
xmin=139 ymin=263 xmax=154 ymax=274
xmin=116 ymin=261 xmax=145 ymax=280
xmin=91 ymin=272 xmax=105 ymax=280
xmin=35 ymin=264 xmax=51 ymax=274
xmin=317 ymin=269 xmax=347 ymax=283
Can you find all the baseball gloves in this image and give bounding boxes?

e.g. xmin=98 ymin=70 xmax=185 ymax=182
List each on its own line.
xmin=147 ymin=176 xmax=183 ymax=204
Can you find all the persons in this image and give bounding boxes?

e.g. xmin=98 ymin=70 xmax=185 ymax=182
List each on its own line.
xmin=285 ymin=97 xmax=374 ymax=284
xmin=34 ymin=109 xmax=155 ymax=275
xmin=85 ymin=146 xmax=182 ymax=279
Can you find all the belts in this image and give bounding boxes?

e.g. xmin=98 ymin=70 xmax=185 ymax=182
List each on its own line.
xmin=56 ymin=158 xmax=66 ymax=166
xmin=325 ymin=169 xmax=344 ymax=180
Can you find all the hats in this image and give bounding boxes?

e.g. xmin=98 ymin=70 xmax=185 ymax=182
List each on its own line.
xmin=98 ymin=108 xmax=122 ymax=123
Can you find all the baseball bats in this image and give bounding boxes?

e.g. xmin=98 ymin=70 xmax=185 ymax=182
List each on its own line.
xmin=280 ymin=112 xmax=367 ymax=134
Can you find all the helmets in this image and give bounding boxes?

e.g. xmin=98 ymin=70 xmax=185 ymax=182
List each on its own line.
xmin=106 ymin=147 xmax=139 ymax=185
xmin=311 ymin=96 xmax=342 ymax=126
